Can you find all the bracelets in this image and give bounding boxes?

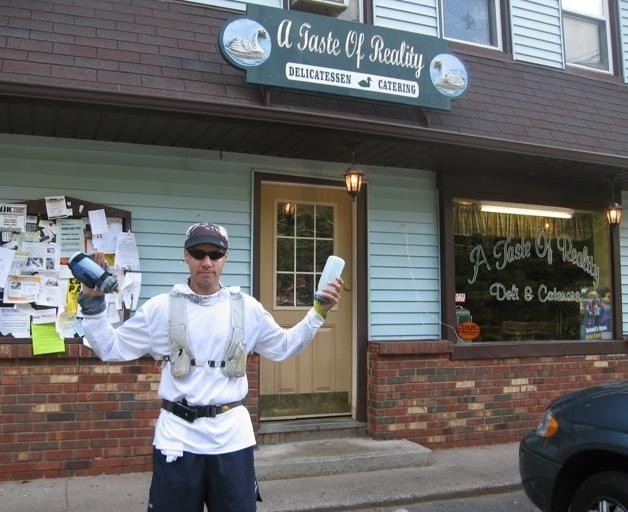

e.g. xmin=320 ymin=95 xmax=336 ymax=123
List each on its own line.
xmin=313 ymin=303 xmax=330 ymax=317
xmin=76 ymin=291 xmax=107 ymax=315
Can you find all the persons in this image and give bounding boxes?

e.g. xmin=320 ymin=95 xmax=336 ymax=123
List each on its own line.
xmin=77 ymin=222 xmax=345 ymax=512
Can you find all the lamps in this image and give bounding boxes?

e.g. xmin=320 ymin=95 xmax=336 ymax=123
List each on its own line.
xmin=603 ymin=174 xmax=625 ymax=228
xmin=282 ymin=203 xmax=295 ymax=221
xmin=342 ymin=151 xmax=367 ymax=203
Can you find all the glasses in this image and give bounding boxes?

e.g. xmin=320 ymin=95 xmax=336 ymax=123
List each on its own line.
xmin=187 ymin=247 xmax=226 ymax=261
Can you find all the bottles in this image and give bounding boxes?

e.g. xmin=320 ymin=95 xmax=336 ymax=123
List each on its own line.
xmin=314 ymin=255 xmax=346 ymax=304
xmin=67 ymin=251 xmax=118 ymax=294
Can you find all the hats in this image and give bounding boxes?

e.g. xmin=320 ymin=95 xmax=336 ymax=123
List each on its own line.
xmin=184 ymin=223 xmax=229 ymax=250
